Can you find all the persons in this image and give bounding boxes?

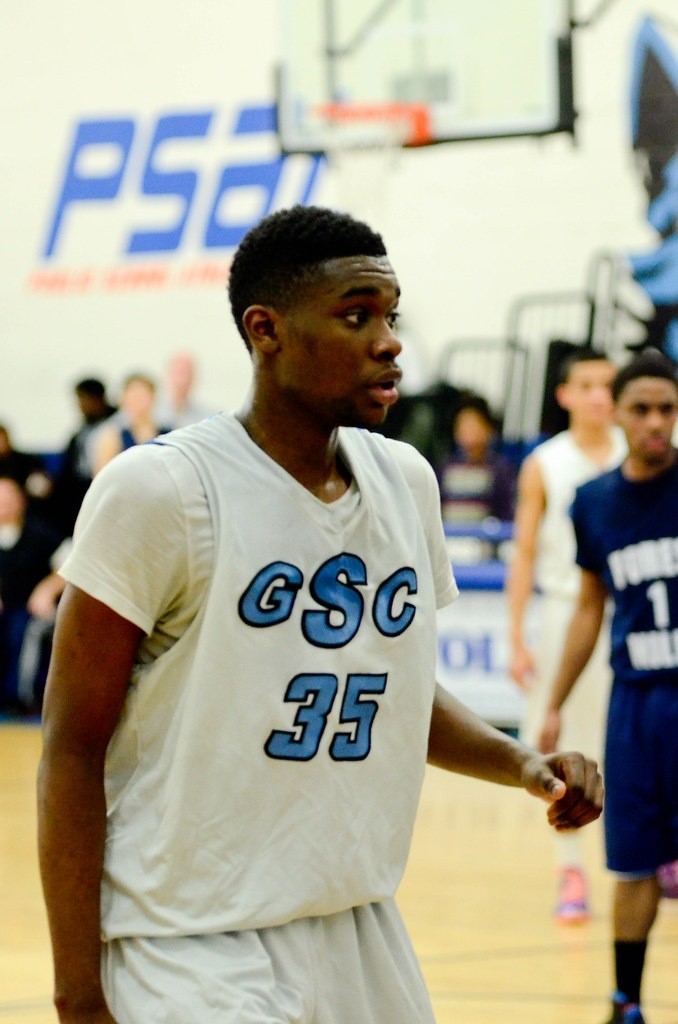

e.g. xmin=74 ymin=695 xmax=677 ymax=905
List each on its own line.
xmin=506 ymin=348 xmax=627 ymax=926
xmin=537 ymin=357 xmax=678 ymax=1024
xmin=0 ymin=353 xmax=200 ymax=720
xmin=33 ymin=208 xmax=603 ymax=1024
xmin=436 ymin=395 xmax=518 ymax=548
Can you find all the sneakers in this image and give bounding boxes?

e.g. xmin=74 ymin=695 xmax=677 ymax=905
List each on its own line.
xmin=553 ymin=868 xmax=589 ymax=924
xmin=607 ymin=1003 xmax=645 ymax=1024
